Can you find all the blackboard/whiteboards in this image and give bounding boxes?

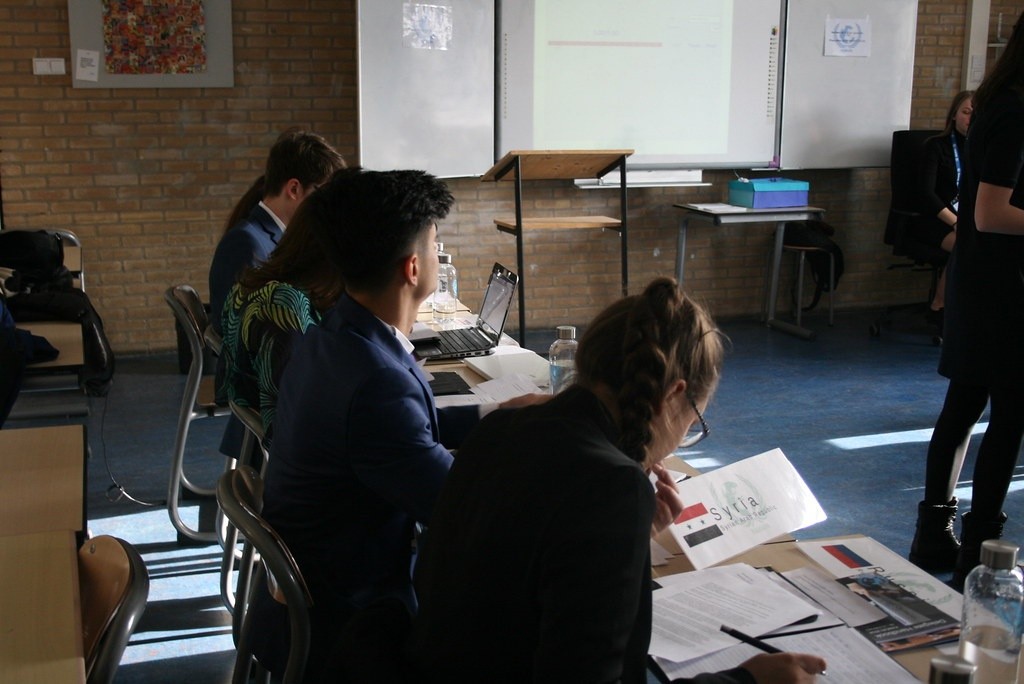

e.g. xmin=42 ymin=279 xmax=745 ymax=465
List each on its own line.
xmin=354 ymin=0 xmax=919 ymax=179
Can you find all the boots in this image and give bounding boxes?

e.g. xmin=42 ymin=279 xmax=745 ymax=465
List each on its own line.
xmin=953 ymin=507 xmax=1009 ymax=592
xmin=909 ymin=494 xmax=962 ymax=575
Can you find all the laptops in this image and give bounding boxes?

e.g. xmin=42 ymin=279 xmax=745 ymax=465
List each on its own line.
xmin=413 ymin=261 xmax=517 ymax=360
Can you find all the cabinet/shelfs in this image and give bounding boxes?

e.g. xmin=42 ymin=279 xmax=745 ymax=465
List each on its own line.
xmin=478 ymin=148 xmax=635 ymax=350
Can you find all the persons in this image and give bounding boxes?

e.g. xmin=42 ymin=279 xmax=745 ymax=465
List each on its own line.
xmin=920 ymin=91 xmax=976 ymax=326
xmin=909 ymin=12 xmax=1024 ymax=586
xmin=208 ymin=132 xmax=348 ymax=335
xmin=261 ymin=169 xmax=556 ymax=684
xmin=214 ymin=175 xmax=344 ymax=476
xmin=412 ymin=277 xmax=828 ymax=684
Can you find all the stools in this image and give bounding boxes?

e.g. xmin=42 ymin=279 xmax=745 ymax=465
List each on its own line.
xmin=759 ymin=239 xmax=836 ymax=326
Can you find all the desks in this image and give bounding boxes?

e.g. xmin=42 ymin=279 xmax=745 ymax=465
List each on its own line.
xmin=423 ymin=348 xmax=577 ymax=410
xmin=0 ymin=528 xmax=103 ymax=684
xmin=413 ymin=312 xmax=522 ymax=365
xmin=16 ymin=322 xmax=84 ymax=373
xmin=671 ymin=200 xmax=828 ymax=345
xmin=417 ymin=289 xmax=473 ymax=312
xmin=0 ymin=423 xmax=92 ymax=535
xmin=643 ymin=532 xmax=1024 ymax=684
xmin=647 ymin=438 xmax=797 ymax=556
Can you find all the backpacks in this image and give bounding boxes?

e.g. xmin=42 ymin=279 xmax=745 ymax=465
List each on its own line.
xmin=0 ymin=227 xmax=86 ymax=325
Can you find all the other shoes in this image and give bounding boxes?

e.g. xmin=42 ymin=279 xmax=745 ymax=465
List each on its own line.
xmin=927 ymin=305 xmax=945 ymax=330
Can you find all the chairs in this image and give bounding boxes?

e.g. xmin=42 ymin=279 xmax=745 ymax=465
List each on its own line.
xmin=867 ymin=130 xmax=951 ymax=348
xmin=212 ymin=460 xmax=316 ymax=684
xmin=194 ymin=316 xmax=281 ymax=552
xmin=43 ymin=228 xmax=85 ymax=291
xmin=158 ymin=283 xmax=246 ymax=547
xmin=217 ymin=378 xmax=291 ymax=665
xmin=75 ymin=531 xmax=154 ymax=684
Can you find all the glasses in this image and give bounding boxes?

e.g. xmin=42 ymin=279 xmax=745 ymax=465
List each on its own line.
xmin=677 ymin=388 xmax=711 ymax=448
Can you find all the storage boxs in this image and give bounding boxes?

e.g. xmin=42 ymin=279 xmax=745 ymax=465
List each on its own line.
xmin=726 ymin=177 xmax=809 ymax=207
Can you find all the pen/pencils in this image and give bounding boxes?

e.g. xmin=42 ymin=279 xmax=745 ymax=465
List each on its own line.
xmin=720 ymin=625 xmax=826 ymax=675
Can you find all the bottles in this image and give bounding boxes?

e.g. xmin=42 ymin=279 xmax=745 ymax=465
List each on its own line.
xmin=928 ymin=654 xmax=978 ymax=683
xmin=425 ymin=242 xmax=458 ymax=324
xmin=548 ymin=324 xmax=579 ymax=389
xmin=956 ymin=536 xmax=1024 ymax=682
